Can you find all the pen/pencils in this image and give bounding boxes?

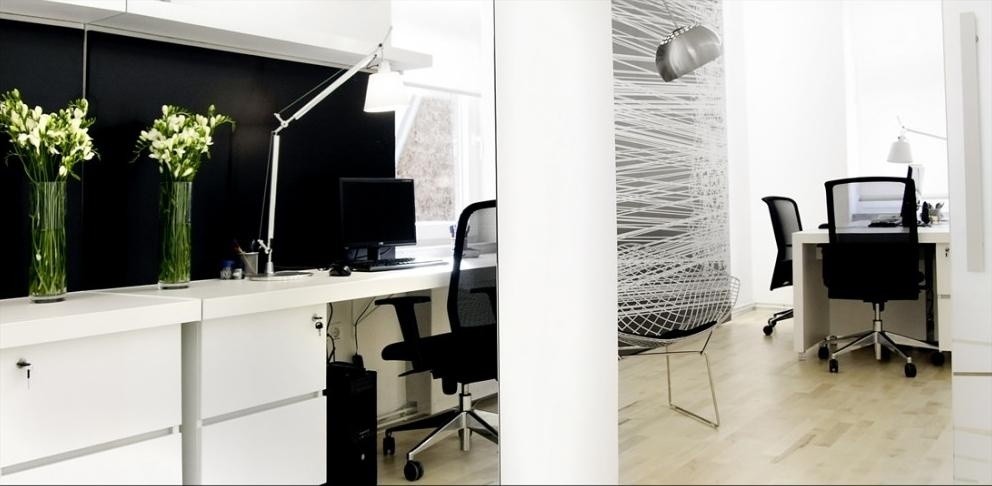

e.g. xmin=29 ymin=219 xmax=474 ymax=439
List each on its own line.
xmin=449 ymin=225 xmax=470 ymax=238
xmin=231 ymin=238 xmax=256 ymax=273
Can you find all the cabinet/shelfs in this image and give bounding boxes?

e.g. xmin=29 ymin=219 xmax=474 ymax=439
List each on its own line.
xmin=0 ymin=318 xmax=202 ymax=485
xmin=183 ymin=296 xmax=329 ymax=486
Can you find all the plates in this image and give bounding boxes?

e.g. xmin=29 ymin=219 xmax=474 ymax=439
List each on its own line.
xmin=462 ymin=249 xmax=480 ymax=258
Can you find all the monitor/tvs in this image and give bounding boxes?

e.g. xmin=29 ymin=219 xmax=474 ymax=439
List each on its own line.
xmin=339 ymin=176 xmax=416 ymax=261
xmin=901 ymin=165 xmax=924 ymax=226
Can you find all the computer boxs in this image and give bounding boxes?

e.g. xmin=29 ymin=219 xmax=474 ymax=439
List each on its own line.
xmin=326 ymin=361 xmax=377 ymax=485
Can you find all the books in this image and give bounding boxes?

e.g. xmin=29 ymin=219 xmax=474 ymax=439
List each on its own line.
xmin=867 ymin=211 xmax=903 ymax=228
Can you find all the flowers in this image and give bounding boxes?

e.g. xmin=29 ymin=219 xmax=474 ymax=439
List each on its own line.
xmin=0 ymin=89 xmax=101 ymax=296
xmin=131 ymin=100 xmax=236 ymax=283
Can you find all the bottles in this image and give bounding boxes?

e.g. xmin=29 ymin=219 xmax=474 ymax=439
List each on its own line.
xmin=220 ymin=259 xmax=234 ymax=279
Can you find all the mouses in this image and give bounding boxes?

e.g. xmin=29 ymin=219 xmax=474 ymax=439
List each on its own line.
xmin=330 ymin=265 xmax=351 ymax=276
xmin=818 ymin=223 xmax=836 ymax=228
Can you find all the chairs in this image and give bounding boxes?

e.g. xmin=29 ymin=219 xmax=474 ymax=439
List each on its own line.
xmin=760 ymin=196 xmax=803 ymax=337
xmin=818 ymin=176 xmax=946 ymax=378
xmin=373 ymin=200 xmax=498 ymax=479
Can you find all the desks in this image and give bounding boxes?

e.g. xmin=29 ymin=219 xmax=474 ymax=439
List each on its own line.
xmin=791 ymin=214 xmax=951 ymax=354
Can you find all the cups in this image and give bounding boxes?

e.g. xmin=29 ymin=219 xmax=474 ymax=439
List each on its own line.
xmin=238 ymin=252 xmax=259 ymax=277
xmin=927 ymin=209 xmax=942 ymax=225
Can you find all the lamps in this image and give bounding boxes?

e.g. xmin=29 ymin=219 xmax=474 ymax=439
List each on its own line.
xmin=247 ymin=44 xmax=412 ymax=284
xmin=655 ymin=1 xmax=722 ymax=82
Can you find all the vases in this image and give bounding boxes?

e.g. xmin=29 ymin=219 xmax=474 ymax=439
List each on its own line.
xmin=27 ymin=180 xmax=68 ymax=304
xmin=157 ymin=180 xmax=194 ymax=287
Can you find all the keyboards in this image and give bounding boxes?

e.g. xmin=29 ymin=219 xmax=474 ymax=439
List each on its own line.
xmin=869 ymin=217 xmax=902 ymax=227
xmin=358 ymin=259 xmax=447 ymax=271
